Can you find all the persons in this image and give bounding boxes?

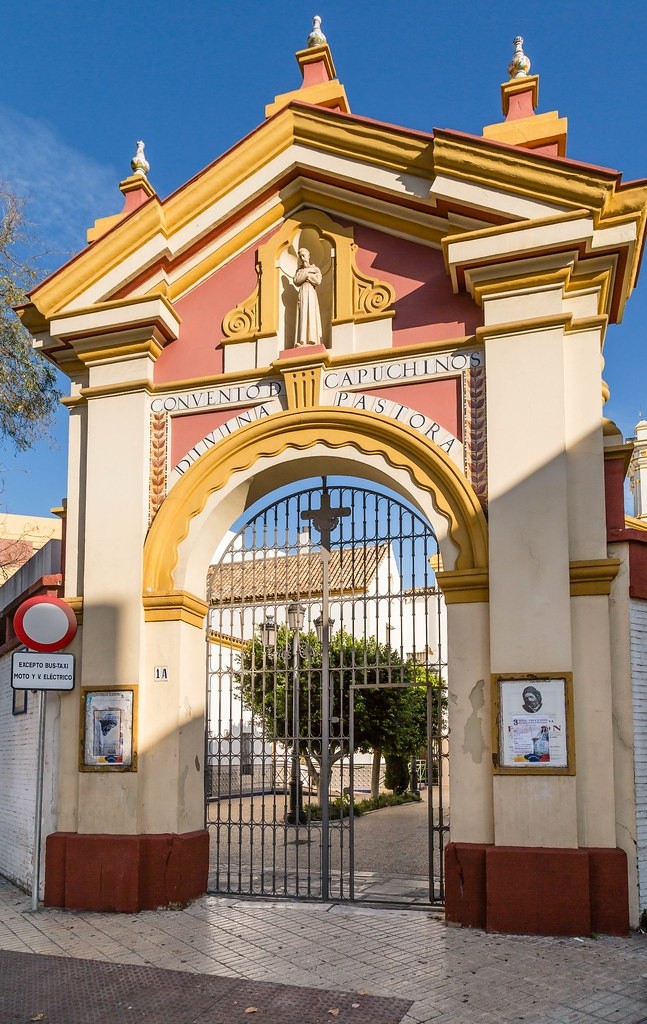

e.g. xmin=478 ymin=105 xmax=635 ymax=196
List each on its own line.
xmin=521 ymin=686 xmax=542 ymax=713
xmin=293 ymin=247 xmax=323 ymax=348
